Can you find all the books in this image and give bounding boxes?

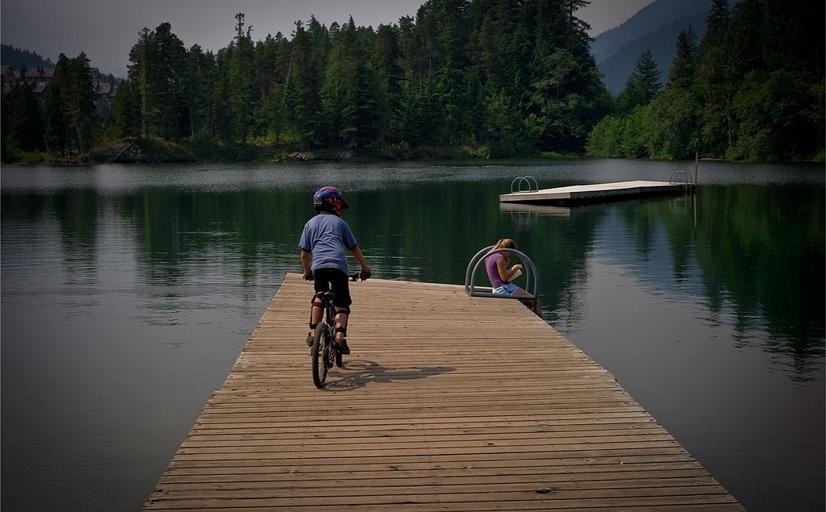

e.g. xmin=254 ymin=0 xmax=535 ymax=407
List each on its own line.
xmin=506 ymin=268 xmax=522 ymax=283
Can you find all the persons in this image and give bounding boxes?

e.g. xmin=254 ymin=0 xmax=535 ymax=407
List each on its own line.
xmin=485 ymin=238 xmax=541 ymax=317
xmin=297 ymin=186 xmax=370 ymax=355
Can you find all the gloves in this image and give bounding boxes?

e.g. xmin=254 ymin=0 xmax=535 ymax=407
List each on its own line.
xmin=360 ymin=271 xmax=370 ymax=281
xmin=304 ymin=271 xmax=313 ymax=280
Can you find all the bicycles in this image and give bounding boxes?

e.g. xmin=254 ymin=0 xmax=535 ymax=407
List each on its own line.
xmin=303 ymin=272 xmax=370 ymax=388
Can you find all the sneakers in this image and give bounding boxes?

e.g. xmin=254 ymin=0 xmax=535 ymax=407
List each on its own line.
xmin=336 ymin=339 xmax=350 ymax=355
xmin=306 ymin=333 xmax=314 ymax=346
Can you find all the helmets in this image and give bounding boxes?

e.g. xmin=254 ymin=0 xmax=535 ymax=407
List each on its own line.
xmin=313 ymin=186 xmax=343 ymax=217
